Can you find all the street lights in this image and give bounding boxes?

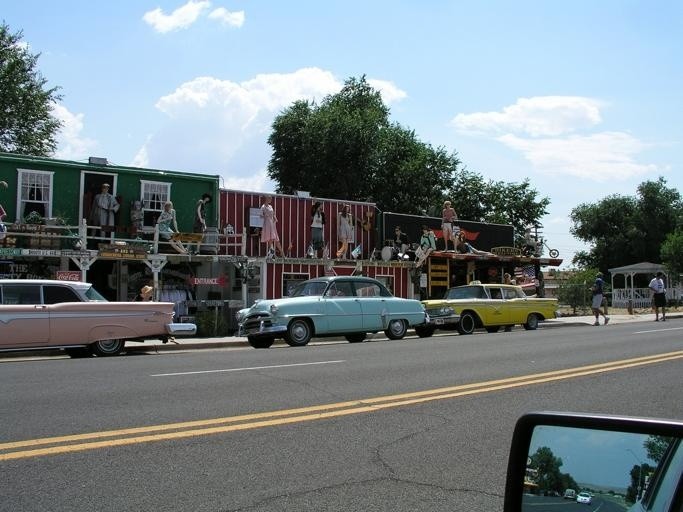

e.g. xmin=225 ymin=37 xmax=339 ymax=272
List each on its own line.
xmin=623 ymin=446 xmax=641 ymax=504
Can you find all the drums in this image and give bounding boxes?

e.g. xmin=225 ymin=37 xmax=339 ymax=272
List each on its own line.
xmin=381 ymin=246 xmax=396 ymax=261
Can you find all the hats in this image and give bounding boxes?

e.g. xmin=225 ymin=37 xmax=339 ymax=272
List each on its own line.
xmin=140 ymin=285 xmax=153 ymax=298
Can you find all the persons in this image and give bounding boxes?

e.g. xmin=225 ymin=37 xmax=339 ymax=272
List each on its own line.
xmin=126 ymin=199 xmax=145 ymax=239
xmin=191 ymin=192 xmax=212 ymax=254
xmin=308 ymin=201 xmax=326 ymax=259
xmin=588 ymin=271 xmax=610 ymax=326
xmin=453 ymin=231 xmax=494 ymax=255
xmin=155 ymin=201 xmax=187 ymax=254
xmin=131 ymin=285 xmax=154 ymax=301
xmin=502 ymin=273 xmax=518 ymax=332
xmin=413 ymin=224 xmax=437 ymax=268
xmin=336 ymin=204 xmax=354 ymax=259
xmin=392 ymin=224 xmax=412 ymax=260
xmin=440 ymin=200 xmax=458 ymax=252
xmin=89 ymin=181 xmax=121 ymax=242
xmin=647 ymin=271 xmax=668 ymax=322
xmin=258 ymin=194 xmax=288 ymax=258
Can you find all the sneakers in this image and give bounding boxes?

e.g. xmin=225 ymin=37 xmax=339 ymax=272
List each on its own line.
xmin=592 ymin=322 xmax=599 ymax=327
xmin=654 ymin=318 xmax=666 ymax=322
xmin=604 ymin=317 xmax=610 ymax=325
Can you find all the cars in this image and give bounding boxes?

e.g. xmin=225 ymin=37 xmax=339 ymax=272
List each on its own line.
xmin=412 ymin=279 xmax=562 ymax=338
xmin=563 ymin=487 xmax=594 ymax=505
xmin=230 ymin=274 xmax=429 ymax=350
xmin=0 ymin=275 xmax=197 ymax=359
